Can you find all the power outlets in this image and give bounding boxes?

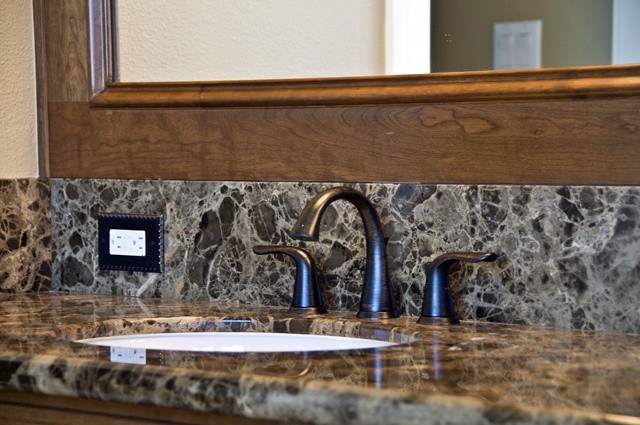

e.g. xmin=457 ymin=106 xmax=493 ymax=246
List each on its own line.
xmin=97 ymin=212 xmax=164 ymax=274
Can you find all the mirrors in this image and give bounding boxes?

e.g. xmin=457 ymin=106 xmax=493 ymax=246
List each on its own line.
xmin=32 ymin=0 xmax=638 ymax=186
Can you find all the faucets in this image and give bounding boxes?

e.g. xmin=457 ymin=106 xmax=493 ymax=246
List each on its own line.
xmin=288 ymin=185 xmax=392 ymax=319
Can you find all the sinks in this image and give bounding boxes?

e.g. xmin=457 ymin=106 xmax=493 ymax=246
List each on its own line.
xmin=71 ymin=332 xmax=400 ymax=354
